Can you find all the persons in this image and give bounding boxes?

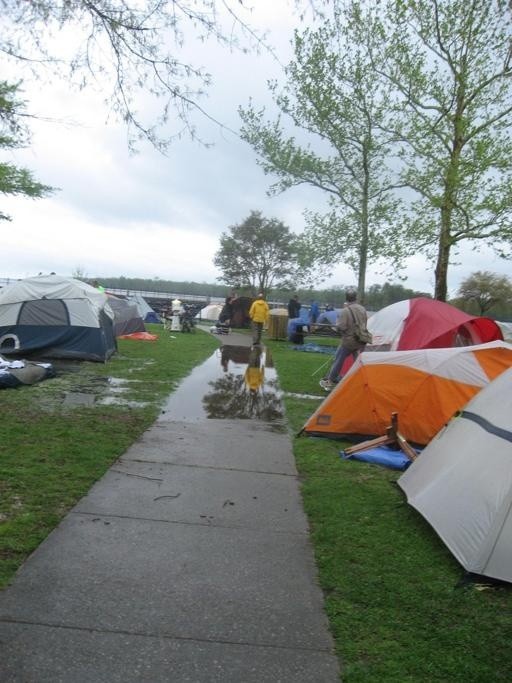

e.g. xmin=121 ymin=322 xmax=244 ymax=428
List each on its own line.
xmin=319 ymin=289 xmax=368 ymax=391
xmin=248 ymin=294 xmax=270 ymax=346
xmin=244 ymin=347 xmax=264 ymax=397
xmin=288 ymin=295 xmax=301 ymax=318
xmin=311 ymin=300 xmax=319 ymax=319
xmin=217 ymin=297 xmax=235 ymax=324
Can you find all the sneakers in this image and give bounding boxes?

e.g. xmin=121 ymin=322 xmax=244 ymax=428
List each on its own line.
xmin=320 ymin=378 xmax=333 ymax=392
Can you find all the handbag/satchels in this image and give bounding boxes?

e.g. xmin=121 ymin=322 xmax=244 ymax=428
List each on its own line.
xmin=347 ymin=307 xmax=372 ymax=344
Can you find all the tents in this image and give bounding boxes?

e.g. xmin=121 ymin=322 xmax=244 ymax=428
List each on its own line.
xmin=0 ymin=356 xmax=56 ymax=388
xmin=219 ymin=296 xmax=254 ymax=328
xmin=193 ymin=303 xmax=222 ymax=320
xmin=0 ymin=274 xmax=118 ymax=363
xmin=294 ymin=340 xmax=511 ymax=435
xmin=312 ymin=310 xmax=340 ymax=337
xmin=334 ymin=297 xmax=504 ymax=379
xmin=396 ymin=361 xmax=512 ymax=579
xmin=98 ymin=286 xmax=146 ymax=335
xmin=123 ymin=295 xmax=162 ymax=324
